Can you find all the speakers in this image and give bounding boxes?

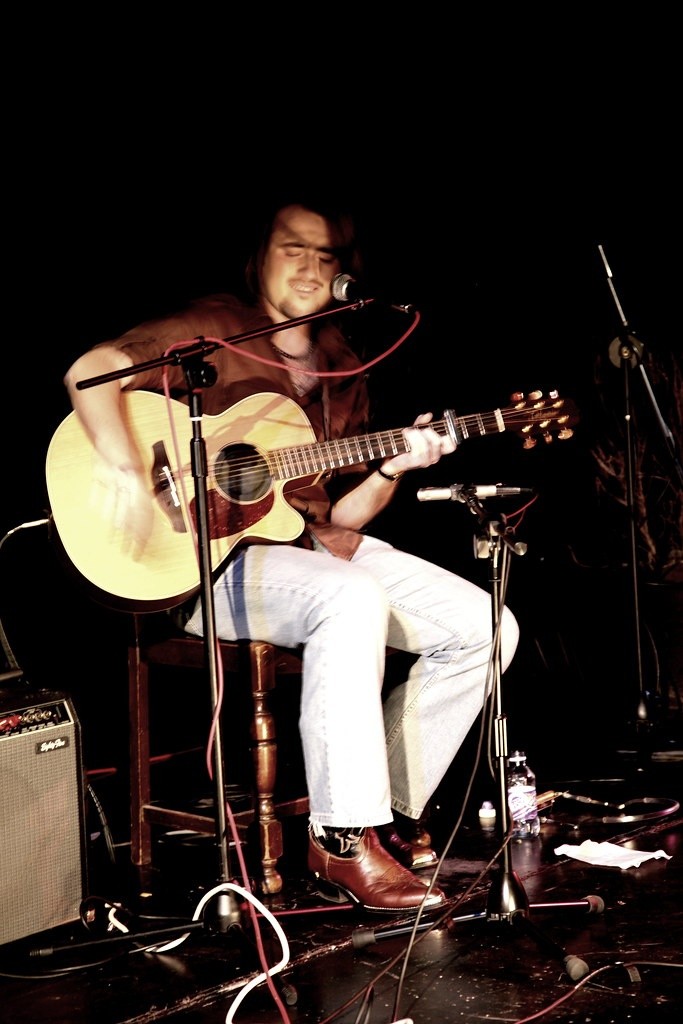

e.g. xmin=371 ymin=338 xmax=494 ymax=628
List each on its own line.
xmin=0 ymin=688 xmax=84 ymax=946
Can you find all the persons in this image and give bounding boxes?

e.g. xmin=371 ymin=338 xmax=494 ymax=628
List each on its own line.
xmin=64 ymin=175 xmax=519 ymax=911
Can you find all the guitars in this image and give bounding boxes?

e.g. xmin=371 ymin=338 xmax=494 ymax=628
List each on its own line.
xmin=44 ymin=386 xmax=585 ymax=617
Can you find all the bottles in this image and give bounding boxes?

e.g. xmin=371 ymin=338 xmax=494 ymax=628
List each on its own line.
xmin=506 ymin=750 xmax=541 ymax=839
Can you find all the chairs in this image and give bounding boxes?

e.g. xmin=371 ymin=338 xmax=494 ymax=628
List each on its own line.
xmin=124 ymin=614 xmax=434 ymax=896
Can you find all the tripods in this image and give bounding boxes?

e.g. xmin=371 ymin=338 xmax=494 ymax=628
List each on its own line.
xmin=28 ymin=299 xmax=609 ymax=1009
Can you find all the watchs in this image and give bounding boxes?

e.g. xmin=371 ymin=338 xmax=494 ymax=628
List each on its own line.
xmin=378 ymin=469 xmax=404 ymax=483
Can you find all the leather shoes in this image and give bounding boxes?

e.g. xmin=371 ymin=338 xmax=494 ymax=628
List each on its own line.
xmin=374 ymin=823 xmax=439 ymax=870
xmin=308 ymin=827 xmax=450 ymax=915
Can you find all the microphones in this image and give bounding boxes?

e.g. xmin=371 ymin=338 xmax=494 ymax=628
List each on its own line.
xmin=331 ymin=274 xmax=414 ymax=315
xmin=416 ymin=485 xmax=534 ymax=501
